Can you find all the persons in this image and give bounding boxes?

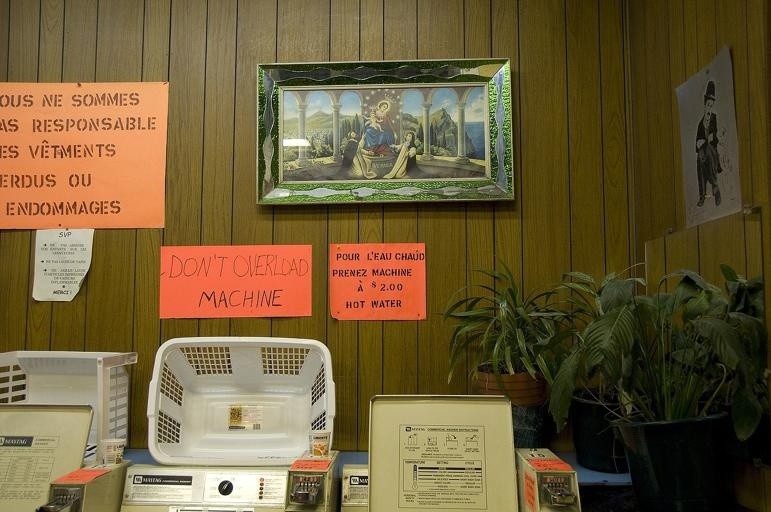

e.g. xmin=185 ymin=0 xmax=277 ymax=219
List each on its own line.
xmin=694 ymin=79 xmax=723 ymax=206
xmin=326 ymin=101 xmax=440 ymax=179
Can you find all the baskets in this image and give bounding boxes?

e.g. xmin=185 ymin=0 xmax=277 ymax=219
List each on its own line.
xmin=146 ymin=336 xmax=335 ymax=468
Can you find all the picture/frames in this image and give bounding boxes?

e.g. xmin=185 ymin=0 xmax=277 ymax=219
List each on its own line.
xmin=252 ymin=54 xmax=517 ymax=207
